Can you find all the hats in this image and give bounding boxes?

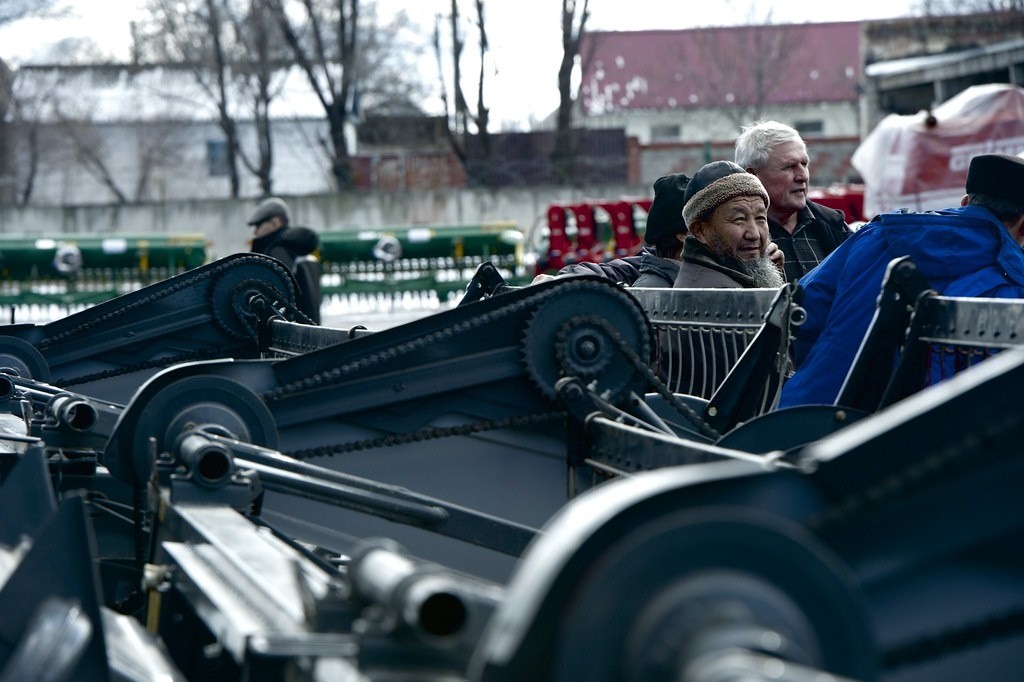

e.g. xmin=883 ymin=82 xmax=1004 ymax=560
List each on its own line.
xmin=682 ymin=161 xmax=769 ymax=231
xmin=644 ymin=173 xmax=691 ymax=245
xmin=248 ymin=197 xmax=289 ymax=226
xmin=966 ymin=155 xmax=1024 ymax=205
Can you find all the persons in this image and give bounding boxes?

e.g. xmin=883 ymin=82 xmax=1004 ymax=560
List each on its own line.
xmin=733 ymin=120 xmax=854 ymax=294
xmin=770 ymin=153 xmax=1024 ymax=411
xmin=630 ymin=172 xmax=691 ymax=343
xmin=247 ymin=196 xmax=321 ymax=326
xmin=657 ymin=161 xmax=786 ymax=401
